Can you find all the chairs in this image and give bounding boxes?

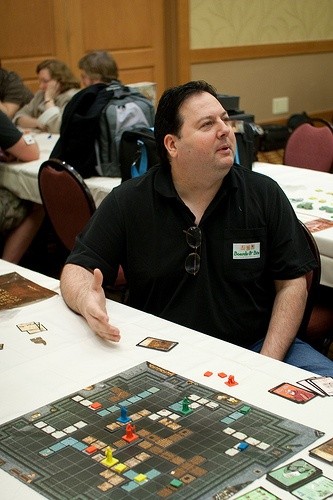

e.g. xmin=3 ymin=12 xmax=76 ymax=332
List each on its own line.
xmin=281 ymin=117 xmax=333 ymax=174
xmin=37 ymin=158 xmax=126 ymax=288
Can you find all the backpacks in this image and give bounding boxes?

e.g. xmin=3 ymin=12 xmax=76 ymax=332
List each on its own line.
xmin=95 ymin=94 xmax=155 ymax=177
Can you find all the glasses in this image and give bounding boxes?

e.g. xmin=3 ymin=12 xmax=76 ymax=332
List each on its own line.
xmin=185 ymin=226 xmax=203 ymax=275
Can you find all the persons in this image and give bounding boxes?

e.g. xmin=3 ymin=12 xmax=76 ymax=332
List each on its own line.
xmin=60 ymin=80 xmax=333 ymax=378
xmin=0 ymin=68 xmax=36 ymax=119
xmin=0 ymin=109 xmax=48 ymax=264
xmin=48 ymin=51 xmax=159 ymax=179
xmin=12 ymin=57 xmax=84 ymax=134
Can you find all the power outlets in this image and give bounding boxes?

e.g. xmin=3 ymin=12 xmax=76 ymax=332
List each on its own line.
xmin=272 ymin=96 xmax=289 ymax=114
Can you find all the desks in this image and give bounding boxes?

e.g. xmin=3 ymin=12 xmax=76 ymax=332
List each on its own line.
xmin=0 ymin=258 xmax=333 ymax=500
xmin=1 ymin=134 xmax=333 ymax=289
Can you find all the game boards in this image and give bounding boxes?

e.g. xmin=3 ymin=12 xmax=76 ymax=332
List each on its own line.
xmin=1 ymin=360 xmax=326 ymax=500
